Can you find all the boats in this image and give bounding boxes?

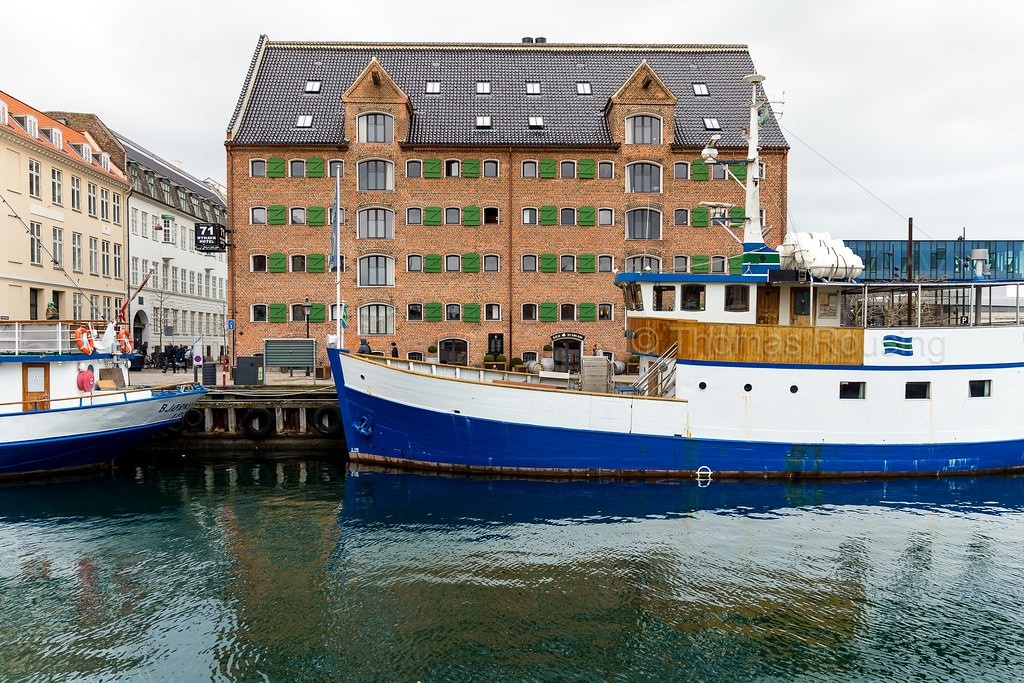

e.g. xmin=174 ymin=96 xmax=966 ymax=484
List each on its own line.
xmin=0 ymin=195 xmax=209 ymax=492
xmin=320 ymin=453 xmax=1024 ymax=683
xmin=323 ymin=61 xmax=1024 ymax=487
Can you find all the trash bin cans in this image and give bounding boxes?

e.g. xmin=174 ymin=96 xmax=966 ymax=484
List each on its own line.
xmin=232 ymin=366 xmax=237 ymax=380
xmin=202 ymin=363 xmax=216 ymax=385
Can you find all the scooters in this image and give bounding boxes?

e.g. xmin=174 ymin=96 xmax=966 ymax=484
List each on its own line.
xmin=141 ymin=352 xmax=170 ymax=369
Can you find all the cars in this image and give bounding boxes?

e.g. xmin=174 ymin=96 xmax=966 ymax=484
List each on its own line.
xmin=173 ymin=348 xmax=207 ymax=368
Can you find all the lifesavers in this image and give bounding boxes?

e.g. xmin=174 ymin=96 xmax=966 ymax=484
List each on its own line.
xmin=245 ymin=409 xmax=274 ymax=438
xmin=75 ymin=326 xmax=95 ymax=355
xmin=118 ymin=330 xmax=135 ymax=354
xmin=169 ymin=408 xmax=204 ymax=430
xmin=314 ymin=404 xmax=342 ymax=435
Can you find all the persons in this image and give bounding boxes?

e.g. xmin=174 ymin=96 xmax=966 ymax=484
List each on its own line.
xmin=357 ymin=338 xmax=369 ymax=354
xmin=390 ymin=342 xmax=399 ymax=358
xmin=176 ymin=344 xmax=187 ymax=373
xmin=136 ymin=340 xmax=147 ymax=357
xmin=162 ymin=341 xmax=176 ymax=373
xmin=592 ymin=343 xmax=603 ymax=356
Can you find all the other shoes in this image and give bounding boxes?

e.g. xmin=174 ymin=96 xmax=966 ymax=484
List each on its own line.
xmin=177 ymin=372 xmax=179 ymax=373
xmin=173 ymin=371 xmax=175 ymax=373
xmin=162 ymin=371 xmax=166 ymax=373
xmin=185 ymin=370 xmax=188 ymax=373
